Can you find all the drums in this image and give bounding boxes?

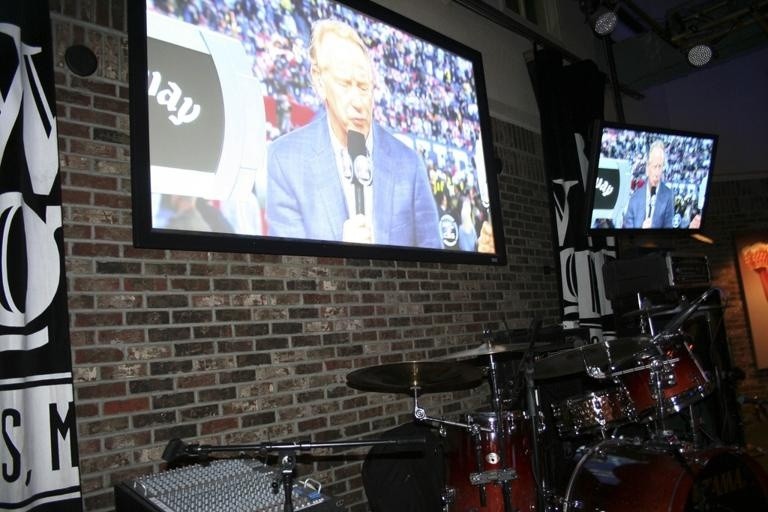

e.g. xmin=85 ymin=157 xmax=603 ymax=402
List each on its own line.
xmin=536 ymin=352 xmax=637 ymax=443
xmin=437 ymin=410 xmax=540 ymax=512
xmin=609 ymin=335 xmax=714 ymax=425
xmin=540 ymin=433 xmax=767 ymax=511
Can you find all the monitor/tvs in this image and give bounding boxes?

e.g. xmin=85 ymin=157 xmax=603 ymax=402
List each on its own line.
xmin=584 ymin=118 xmax=720 ymax=236
xmin=127 ymin=0 xmax=508 ymax=265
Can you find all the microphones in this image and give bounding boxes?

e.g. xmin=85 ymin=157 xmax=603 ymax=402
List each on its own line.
xmin=648 ymin=186 xmax=657 ymax=218
xmin=346 ymin=127 xmax=374 ymax=221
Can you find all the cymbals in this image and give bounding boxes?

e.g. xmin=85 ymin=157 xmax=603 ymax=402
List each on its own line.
xmin=346 ymin=360 xmax=487 ymax=393
xmin=430 ymin=341 xmax=552 ymax=364
xmin=533 ymin=337 xmax=658 ymax=379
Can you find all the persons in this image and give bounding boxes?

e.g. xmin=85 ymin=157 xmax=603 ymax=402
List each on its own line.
xmin=147 ymin=0 xmax=495 ymax=255
xmin=592 ymin=129 xmax=713 ymax=228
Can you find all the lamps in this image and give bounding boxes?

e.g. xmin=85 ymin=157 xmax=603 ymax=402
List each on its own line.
xmin=580 ymin=1 xmax=619 ymax=38
xmin=687 ymin=44 xmax=712 ymax=67
